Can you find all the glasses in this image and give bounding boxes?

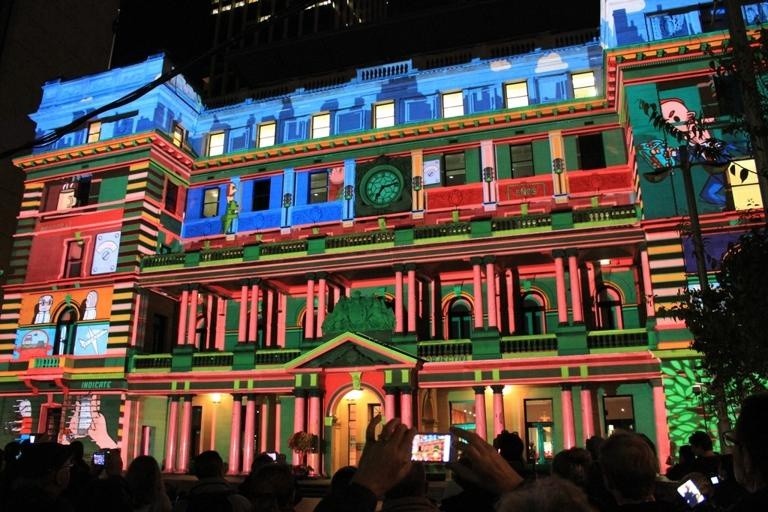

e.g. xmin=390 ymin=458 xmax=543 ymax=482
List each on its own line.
xmin=722 ymin=430 xmax=747 ymax=453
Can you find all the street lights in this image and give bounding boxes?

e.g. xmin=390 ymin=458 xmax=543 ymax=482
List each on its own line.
xmin=644 ymin=145 xmax=735 ymax=453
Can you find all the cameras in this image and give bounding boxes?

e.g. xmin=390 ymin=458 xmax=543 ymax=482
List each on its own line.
xmin=266 ymin=451 xmax=277 ymax=462
xmin=408 ymin=432 xmax=458 ymax=465
xmin=710 ymin=476 xmax=721 ymax=485
xmin=676 ymin=478 xmax=707 ymax=509
xmin=93 ymin=452 xmax=106 ymax=467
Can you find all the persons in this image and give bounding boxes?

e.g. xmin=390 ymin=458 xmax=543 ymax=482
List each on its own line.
xmin=0 ymin=391 xmax=768 ymax=512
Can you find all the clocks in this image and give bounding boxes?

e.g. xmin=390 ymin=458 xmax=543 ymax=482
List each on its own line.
xmin=358 ymin=163 xmax=405 ymax=210
